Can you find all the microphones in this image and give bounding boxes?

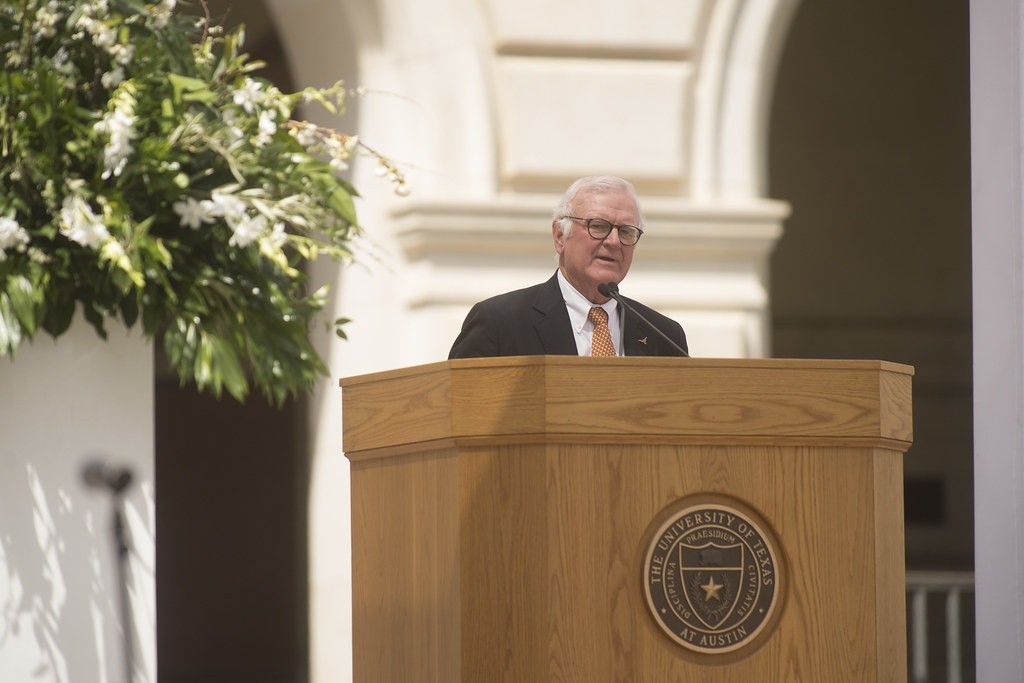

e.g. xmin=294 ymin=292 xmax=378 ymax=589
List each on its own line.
xmin=597 ymin=283 xmax=625 ymax=357
xmin=608 ymin=282 xmax=691 ymax=357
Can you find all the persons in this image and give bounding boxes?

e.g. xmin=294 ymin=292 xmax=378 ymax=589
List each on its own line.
xmin=448 ymin=174 xmax=689 ymax=359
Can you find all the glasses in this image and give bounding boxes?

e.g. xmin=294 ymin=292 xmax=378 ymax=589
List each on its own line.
xmin=561 ymin=216 xmax=644 ymax=246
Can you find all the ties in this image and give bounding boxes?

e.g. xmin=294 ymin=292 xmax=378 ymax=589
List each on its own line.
xmin=588 ymin=307 xmax=617 ymax=357
